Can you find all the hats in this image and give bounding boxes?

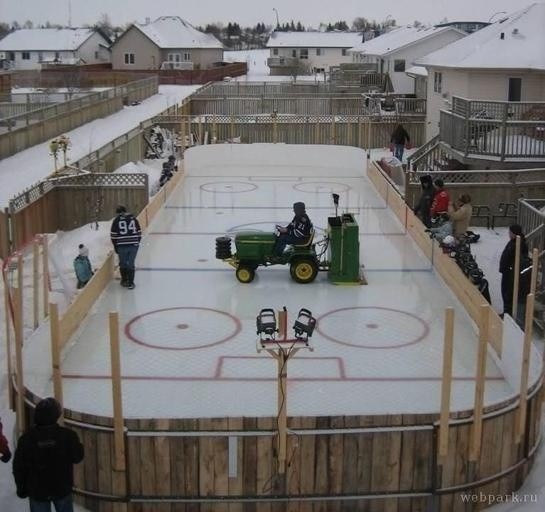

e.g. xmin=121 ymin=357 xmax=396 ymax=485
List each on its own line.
xmin=459 ymin=192 xmax=470 ymax=203
xmin=34 ymin=397 xmax=62 ymax=424
xmin=79 ymin=244 xmax=88 ymax=255
xmin=510 ymin=224 xmax=522 ymax=235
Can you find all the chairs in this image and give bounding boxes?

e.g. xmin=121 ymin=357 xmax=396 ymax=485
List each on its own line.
xmin=293 ymin=230 xmax=316 ymax=249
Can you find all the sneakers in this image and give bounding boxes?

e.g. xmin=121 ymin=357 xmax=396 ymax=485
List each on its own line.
xmin=120 ymin=279 xmax=135 ymax=289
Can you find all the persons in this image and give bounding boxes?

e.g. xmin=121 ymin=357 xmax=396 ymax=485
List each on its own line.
xmin=168 ymin=155 xmax=177 ymax=171
xmin=265 ymin=202 xmax=313 ymax=263
xmin=160 ymin=163 xmax=173 ymax=187
xmin=74 ymin=244 xmax=95 ymax=289
xmin=13 ymin=398 xmax=84 ymax=512
xmin=391 ymin=124 xmax=410 ymax=161
xmin=111 ymin=205 xmax=141 ymax=289
xmin=0 ymin=417 xmax=11 ymax=463
xmin=413 ymin=175 xmax=528 ymax=318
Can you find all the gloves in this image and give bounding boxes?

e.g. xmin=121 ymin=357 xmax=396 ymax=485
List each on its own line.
xmin=0 ymin=449 xmax=11 ymax=463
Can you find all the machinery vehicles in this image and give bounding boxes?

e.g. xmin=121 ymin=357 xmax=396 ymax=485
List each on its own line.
xmin=215 ymin=224 xmax=329 ymax=283
